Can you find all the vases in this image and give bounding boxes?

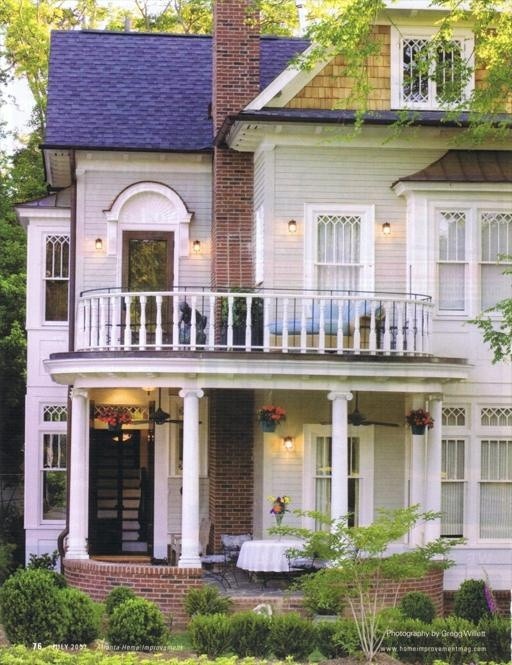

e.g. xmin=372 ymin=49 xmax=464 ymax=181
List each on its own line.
xmin=259 ymin=420 xmax=276 ymax=433
xmin=411 ymin=424 xmax=426 ymax=436
xmin=107 ymin=420 xmax=122 ymax=431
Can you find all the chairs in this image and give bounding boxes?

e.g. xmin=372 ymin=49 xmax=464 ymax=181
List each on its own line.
xmin=167 ymin=519 xmax=253 ymax=591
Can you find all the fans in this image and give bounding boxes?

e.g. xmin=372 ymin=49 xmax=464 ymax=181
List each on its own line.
xmin=127 ymin=387 xmax=201 ymax=425
xmin=320 ymin=392 xmax=400 ymax=427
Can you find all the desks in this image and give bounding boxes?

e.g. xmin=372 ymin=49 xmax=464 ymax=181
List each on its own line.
xmin=235 ymin=540 xmax=321 ymax=592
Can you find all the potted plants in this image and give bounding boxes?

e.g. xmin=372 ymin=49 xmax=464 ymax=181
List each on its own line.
xmin=221 ymin=286 xmax=264 ymax=353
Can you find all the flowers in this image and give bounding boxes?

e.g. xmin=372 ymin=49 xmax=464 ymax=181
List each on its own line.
xmin=404 ymin=408 xmax=433 ymax=430
xmin=96 ymin=406 xmax=131 ymax=427
xmin=255 ymin=406 xmax=287 ymax=423
xmin=265 ymin=495 xmax=290 ymax=527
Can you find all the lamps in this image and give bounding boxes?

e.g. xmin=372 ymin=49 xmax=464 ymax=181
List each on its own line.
xmin=94 ymin=238 xmax=101 ymax=249
xmin=382 ymin=222 xmax=391 ymax=236
xmin=192 ymin=239 xmax=200 ymax=252
xmin=283 ymin=436 xmax=293 ymax=450
xmin=288 ymin=218 xmax=296 ymax=234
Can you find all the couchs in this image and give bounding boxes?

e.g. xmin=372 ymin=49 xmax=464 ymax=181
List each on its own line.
xmin=266 ymin=303 xmax=385 ymax=359
